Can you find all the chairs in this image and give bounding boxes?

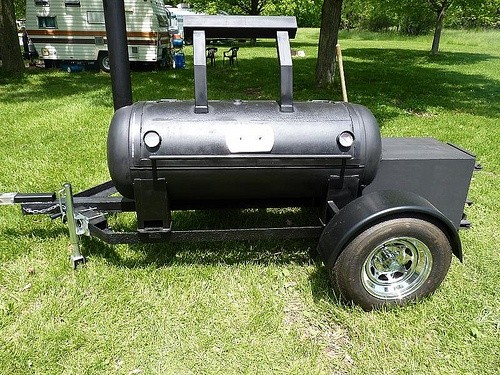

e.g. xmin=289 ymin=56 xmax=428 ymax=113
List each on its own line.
xmin=206 ymin=47 xmax=217 ymax=67
xmin=223 ymin=47 xmax=239 ymax=67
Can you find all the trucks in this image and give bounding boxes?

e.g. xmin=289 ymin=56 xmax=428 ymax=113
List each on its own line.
xmin=164 ymin=3 xmax=230 ymax=44
xmin=26 ymin=0 xmax=177 ymax=71
